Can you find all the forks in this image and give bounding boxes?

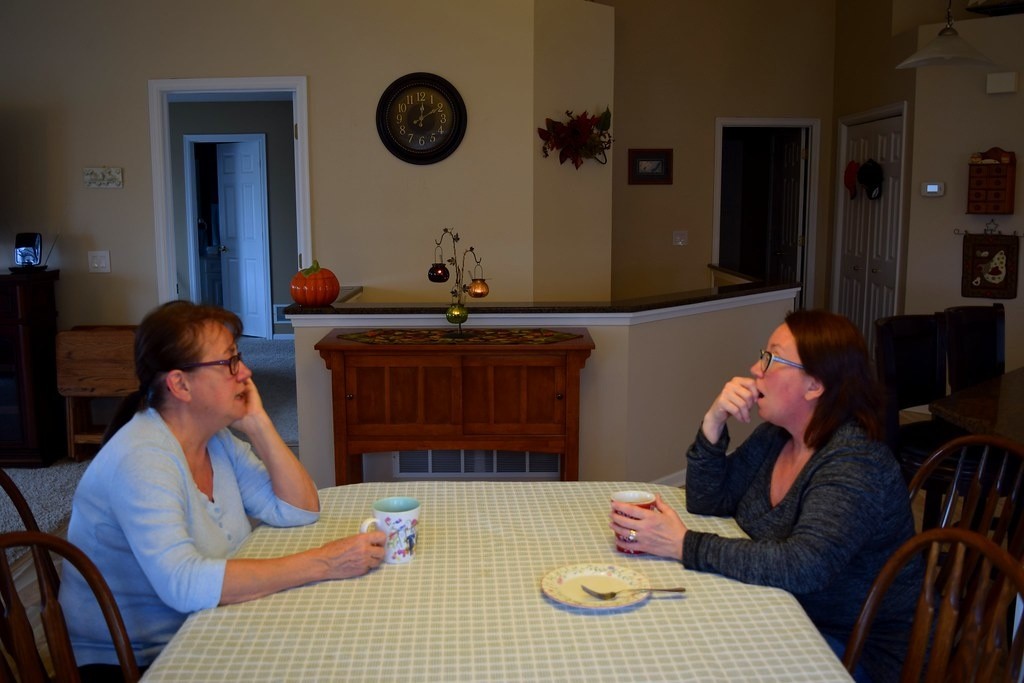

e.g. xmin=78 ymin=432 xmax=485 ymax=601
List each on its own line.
xmin=580 ymin=585 xmax=687 ymax=601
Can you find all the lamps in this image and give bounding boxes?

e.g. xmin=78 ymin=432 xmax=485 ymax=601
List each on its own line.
xmin=895 ymin=0 xmax=997 ymax=71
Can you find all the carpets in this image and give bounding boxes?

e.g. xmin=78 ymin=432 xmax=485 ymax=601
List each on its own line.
xmin=0 ymin=337 xmax=299 ymax=561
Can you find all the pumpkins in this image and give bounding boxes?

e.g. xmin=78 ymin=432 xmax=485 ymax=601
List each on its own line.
xmin=291 ymin=260 xmax=339 ymax=306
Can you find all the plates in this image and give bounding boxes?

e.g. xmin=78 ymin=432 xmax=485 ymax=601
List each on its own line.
xmin=541 ymin=562 xmax=651 ymax=610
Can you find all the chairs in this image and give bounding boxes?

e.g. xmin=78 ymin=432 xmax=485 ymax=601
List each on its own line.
xmin=843 ymin=303 xmax=1024 ymax=683
xmin=0 ymin=530 xmax=141 ymax=683
xmin=0 ymin=469 xmax=61 ymax=654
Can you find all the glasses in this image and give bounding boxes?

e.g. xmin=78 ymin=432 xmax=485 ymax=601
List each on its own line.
xmin=179 ymin=352 xmax=242 ymax=374
xmin=758 ymin=349 xmax=806 ymax=373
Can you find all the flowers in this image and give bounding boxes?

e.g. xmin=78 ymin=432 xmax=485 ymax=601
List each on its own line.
xmin=538 ymin=103 xmax=616 ymax=170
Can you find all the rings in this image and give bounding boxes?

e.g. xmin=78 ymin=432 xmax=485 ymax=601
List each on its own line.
xmin=629 ymin=529 xmax=638 ymax=543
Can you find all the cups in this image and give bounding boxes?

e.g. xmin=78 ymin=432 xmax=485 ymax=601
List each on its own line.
xmin=360 ymin=496 xmax=421 ymax=564
xmin=610 ymin=492 xmax=655 ymax=554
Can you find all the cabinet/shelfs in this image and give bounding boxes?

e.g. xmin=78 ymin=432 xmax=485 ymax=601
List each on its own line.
xmin=0 ymin=269 xmax=62 ymax=469
xmin=314 ymin=328 xmax=595 ymax=483
xmin=965 ymin=146 xmax=1017 ymax=216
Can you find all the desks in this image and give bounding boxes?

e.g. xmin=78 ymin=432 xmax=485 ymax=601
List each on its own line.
xmin=54 ymin=325 xmax=141 ymax=463
xmin=140 ymin=481 xmax=855 ymax=683
xmin=928 ymin=365 xmax=1024 ymax=450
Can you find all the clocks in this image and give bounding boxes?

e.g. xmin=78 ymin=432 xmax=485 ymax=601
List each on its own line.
xmin=376 ymin=71 xmax=467 ymax=165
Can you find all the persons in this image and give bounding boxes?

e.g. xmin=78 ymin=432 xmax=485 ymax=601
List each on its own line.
xmin=58 ymin=298 xmax=386 ymax=683
xmin=609 ymin=309 xmax=938 ymax=683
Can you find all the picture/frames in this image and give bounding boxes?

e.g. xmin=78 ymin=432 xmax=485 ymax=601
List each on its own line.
xmin=628 ymin=149 xmax=674 ymax=185
